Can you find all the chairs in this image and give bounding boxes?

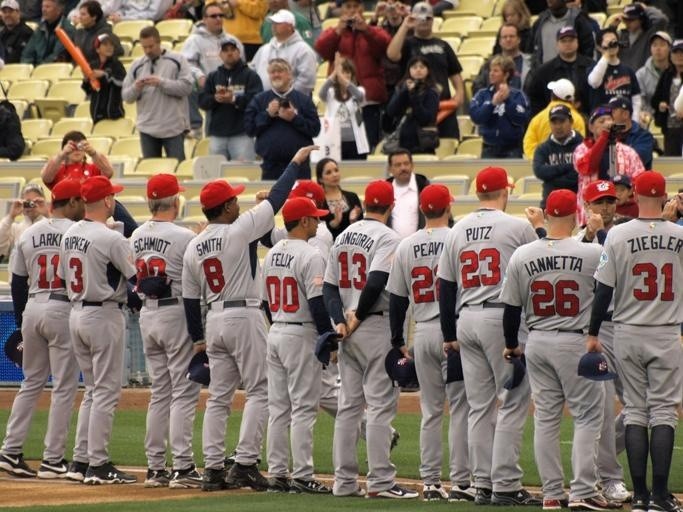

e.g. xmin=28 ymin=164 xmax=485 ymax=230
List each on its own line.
xmin=468 ymin=174 xmax=513 ymax=195
xmin=115 ymin=195 xmax=145 ymax=202
xmin=123 ymin=100 xmax=138 ymax=119
xmin=456 ymin=115 xmax=474 ymax=140
xmin=435 ymin=138 xmax=458 ymax=156
xmin=664 ymin=171 xmax=683 ymax=193
xmin=311 ymin=83 xmax=324 ymax=114
xmin=456 ymin=138 xmax=483 ymax=155
xmin=74 ymin=102 xmax=92 ymax=119
xmin=441 ymin=0 xmax=497 ymax=18
xmin=155 ymin=19 xmax=192 ymax=39
xmin=194 ymin=138 xmax=210 ymax=156
xmin=31 ymin=140 xmax=62 ymax=157
xmin=0 ymin=63 xmax=33 ymax=81
xmin=176 ymin=156 xmax=191 ymax=175
xmin=20 ymin=119 xmax=53 ymax=136
xmin=46 ymin=81 xmax=88 ymax=103
xmin=511 ymin=177 xmax=543 ymax=195
xmin=50 ymin=119 xmax=91 ymax=138
xmin=429 ymin=175 xmax=468 ymax=195
xmin=10 ymin=99 xmax=27 ymax=119
xmin=137 ymin=158 xmax=178 ymax=177
xmin=432 ymin=17 xmax=443 ymax=33
xmin=184 ymin=136 xmax=195 ymax=157
xmin=455 ymin=37 xmax=496 ymax=56
xmin=315 ymin=62 xmax=328 ymax=91
xmin=91 ymin=118 xmax=134 ymax=138
xmin=28 ymin=178 xmax=51 ymax=202
xmin=112 ymin=20 xmax=154 ymax=41
xmin=216 ymin=177 xmax=250 ymax=186
xmin=361 ymin=12 xmax=384 ymax=24
xmin=468 ymin=18 xmax=504 ymax=37
xmin=448 ymin=56 xmax=484 ymax=98
xmin=1 ymin=175 xmax=24 ymax=197
xmin=110 ymin=138 xmax=142 ymax=158
xmin=30 ymin=62 xmax=73 ymax=83
xmin=35 ymin=98 xmax=68 ymax=119
xmin=8 ymin=81 xmax=48 ymax=103
xmin=588 ymin=13 xmax=607 ymax=28
xmin=178 ymin=195 xmax=186 ymax=217
xmin=320 ymin=18 xmax=337 ymax=31
xmin=441 ymin=36 xmax=460 ymax=51
xmin=173 ymin=42 xmax=183 ymax=51
xmin=131 ymin=41 xmax=172 ymax=57
xmin=117 ymin=37 xmax=132 ymax=59
xmin=343 ymin=176 xmax=369 ymax=195
xmin=87 ymin=138 xmax=112 ymax=154
xmin=436 ymin=18 xmax=482 ymax=37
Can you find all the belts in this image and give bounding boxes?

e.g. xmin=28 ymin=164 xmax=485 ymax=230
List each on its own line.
xmin=462 ymin=302 xmax=505 ymax=309
xmin=72 ymin=301 xmax=124 ymax=309
xmin=28 ymin=292 xmax=70 ymax=302
xmin=287 ymin=322 xmax=302 ymax=326
xmin=529 ymin=327 xmax=583 ymax=334
xmin=142 ymin=298 xmax=179 ymax=306
xmin=207 ymin=300 xmax=264 ymax=309
xmin=368 ymin=311 xmax=383 ymax=315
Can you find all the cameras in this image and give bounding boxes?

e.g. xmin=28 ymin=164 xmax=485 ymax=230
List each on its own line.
xmin=609 ymin=39 xmax=630 ymax=50
xmin=346 ymin=18 xmax=356 ymax=25
xmin=385 ymin=5 xmax=396 ymax=12
xmin=278 ymin=97 xmax=289 ymax=108
xmin=23 ymin=201 xmax=36 ymax=208
xmin=417 ymin=15 xmax=430 ymax=25
xmin=72 ymin=143 xmax=84 ymax=151
xmin=610 ymin=123 xmax=626 ymax=145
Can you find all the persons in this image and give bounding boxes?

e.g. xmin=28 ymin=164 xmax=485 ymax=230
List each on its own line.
xmin=0 ymin=0 xmax=683 ymax=237
xmin=1 ymin=167 xmax=683 ymax=512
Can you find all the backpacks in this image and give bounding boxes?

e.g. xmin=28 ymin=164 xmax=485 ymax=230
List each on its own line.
xmin=0 ymin=84 xmax=25 ymax=161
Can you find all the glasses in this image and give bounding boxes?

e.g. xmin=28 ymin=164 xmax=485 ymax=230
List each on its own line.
xmin=592 ymin=198 xmax=616 ymax=204
xmin=206 ymin=13 xmax=224 ymax=18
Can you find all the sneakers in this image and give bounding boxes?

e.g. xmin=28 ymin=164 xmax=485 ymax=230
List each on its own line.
xmin=267 ymin=477 xmax=301 ymax=494
xmin=67 ymin=462 xmax=89 ymax=481
xmin=288 ymin=479 xmax=332 ymax=495
xmin=364 ymin=428 xmax=399 ymax=463
xmin=333 ymin=486 xmax=366 ymax=497
xmin=168 ymin=463 xmax=203 ymax=489
xmin=602 ymin=480 xmax=632 ymax=503
xmin=448 ymin=485 xmax=475 ymax=503
xmin=422 ymin=483 xmax=448 ymax=502
xmin=367 ymin=484 xmax=420 ymax=499
xmin=143 ymin=468 xmax=170 ymax=488
xmin=0 ymin=453 xmax=37 ymax=477
xmin=542 ymin=495 xmax=569 ymax=510
xmin=187 ymin=128 xmax=201 ymax=139
xmin=491 ymin=489 xmax=543 ymax=506
xmin=201 ymin=467 xmax=240 ymax=491
xmin=650 ymin=490 xmax=682 ymax=512
xmin=224 ymin=459 xmax=268 ymax=492
xmin=474 ymin=488 xmax=492 ymax=504
xmin=631 ymin=491 xmax=650 ymax=512
xmin=38 ymin=459 xmax=69 ymax=478
xmin=84 ymin=460 xmax=137 ymax=484
xmin=568 ymin=494 xmax=624 ymax=511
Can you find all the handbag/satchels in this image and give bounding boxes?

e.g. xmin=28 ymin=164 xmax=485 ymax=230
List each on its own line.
xmin=418 ymin=127 xmax=438 ymax=147
xmin=379 ymin=130 xmax=401 ymax=153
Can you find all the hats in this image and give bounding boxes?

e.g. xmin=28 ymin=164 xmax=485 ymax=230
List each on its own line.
xmin=315 ymin=331 xmax=344 ymax=370
xmin=631 ymin=171 xmax=665 ymax=196
xmin=268 ymin=9 xmax=294 ymax=25
xmin=412 ymin=2 xmax=433 ymax=18
xmin=185 ymin=350 xmax=210 ymax=385
xmin=139 ymin=276 xmax=171 ymax=299
xmin=3 ymin=330 xmax=23 ymax=369
xmin=549 ymin=105 xmax=570 ymax=121
xmin=126 ymin=292 xmax=142 ymax=314
xmin=546 ymin=79 xmax=575 ymax=102
xmin=364 ymin=180 xmax=394 ymax=207
xmin=79 ymin=176 xmax=124 ymax=203
xmin=93 ymin=32 xmax=113 ymax=48
xmin=648 ymin=31 xmax=672 ymax=45
xmin=50 ymin=177 xmax=81 ymax=201
xmin=577 ymin=351 xmax=619 ymax=381
xmin=672 ymin=39 xmax=682 ymax=50
xmin=218 ymin=37 xmax=238 ymax=50
xmin=145 ymin=173 xmax=186 ymax=200
xmin=444 ymin=348 xmax=464 ymax=384
xmin=288 ymin=179 xmax=326 ymax=203
xmin=555 ymin=26 xmax=578 ymax=39
xmin=502 ymin=352 xmax=526 ymax=390
xmin=583 ymin=179 xmax=619 ymax=202
xmin=475 ymin=165 xmax=515 ymax=193
xmin=610 ymin=174 xmax=631 ymax=189
xmin=620 ymin=4 xmax=645 ymax=21
xmin=603 ymin=95 xmax=631 ymax=110
xmin=199 ymin=179 xmax=245 ymax=209
xmin=384 ymin=347 xmax=419 ymax=388
xmin=420 ymin=183 xmax=454 ymax=214
xmin=282 ymin=197 xmax=330 ymax=225
xmin=0 ymin=0 xmax=19 ymax=11
xmin=545 ymin=189 xmax=581 ymax=218
